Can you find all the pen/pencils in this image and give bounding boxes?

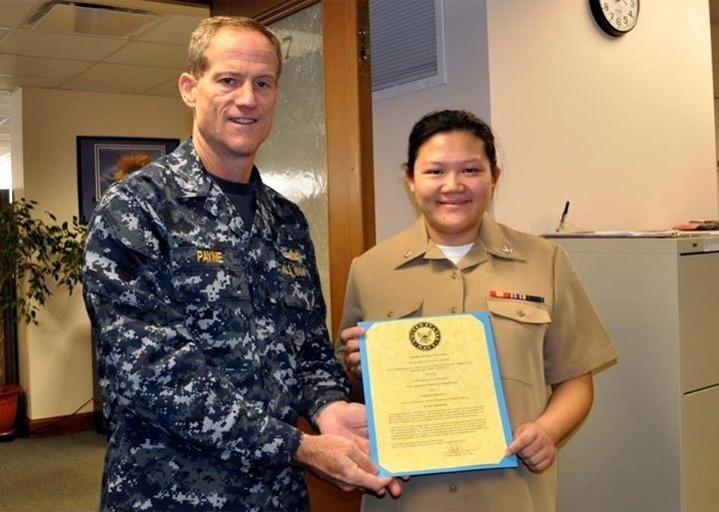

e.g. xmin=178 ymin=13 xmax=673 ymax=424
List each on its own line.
xmin=558 ymin=201 xmax=569 ymax=230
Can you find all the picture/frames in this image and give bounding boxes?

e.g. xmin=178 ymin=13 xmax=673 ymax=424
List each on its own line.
xmin=76 ymin=136 xmax=181 ymax=225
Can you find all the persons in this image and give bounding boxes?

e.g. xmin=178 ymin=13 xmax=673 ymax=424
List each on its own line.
xmin=334 ymin=108 xmax=619 ymax=512
xmin=81 ymin=16 xmax=411 ymax=512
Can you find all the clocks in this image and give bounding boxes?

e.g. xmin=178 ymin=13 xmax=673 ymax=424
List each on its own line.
xmin=590 ymin=0 xmax=641 ymax=37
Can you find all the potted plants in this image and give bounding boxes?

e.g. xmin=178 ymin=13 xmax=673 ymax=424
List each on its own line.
xmin=0 ymin=192 xmax=88 ymax=442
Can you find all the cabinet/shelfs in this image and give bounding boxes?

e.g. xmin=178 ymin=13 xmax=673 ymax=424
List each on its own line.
xmin=547 ymin=234 xmax=719 ymax=512
